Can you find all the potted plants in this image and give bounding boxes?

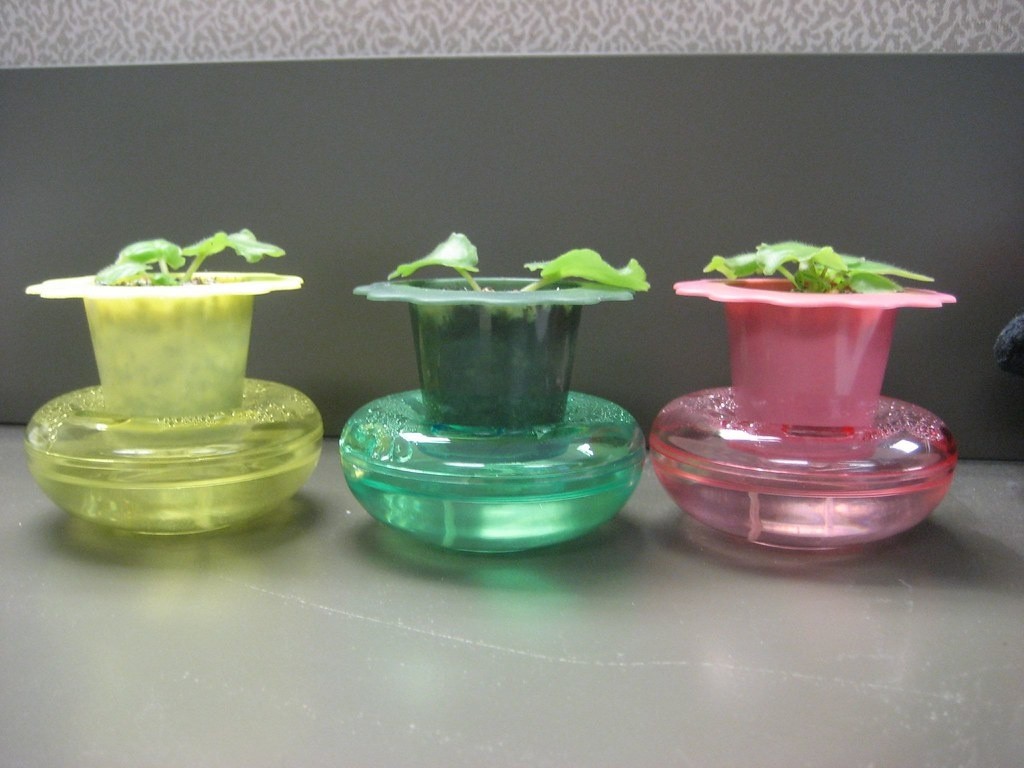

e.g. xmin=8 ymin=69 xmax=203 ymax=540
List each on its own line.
xmin=650 ymin=241 xmax=956 ymax=552
xmin=22 ymin=228 xmax=324 ymax=538
xmin=338 ymin=232 xmax=648 ymax=555
